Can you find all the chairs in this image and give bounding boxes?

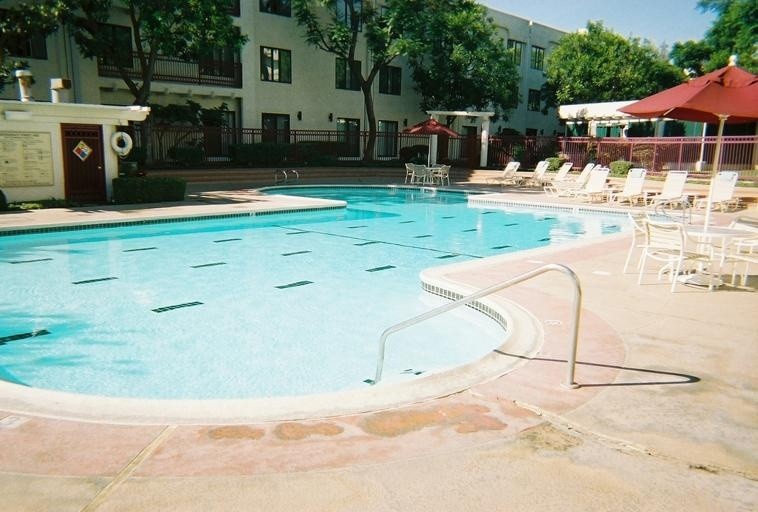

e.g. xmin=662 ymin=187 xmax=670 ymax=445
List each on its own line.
xmin=608 ymin=168 xmax=758 ymax=293
xmin=405 ymin=162 xmax=452 ymax=186
xmin=486 ymin=161 xmax=612 ymax=204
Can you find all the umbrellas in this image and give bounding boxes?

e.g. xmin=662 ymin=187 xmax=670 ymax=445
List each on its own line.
xmin=616 ymin=54 xmax=758 ymax=276
xmin=403 ymin=117 xmax=459 ymax=168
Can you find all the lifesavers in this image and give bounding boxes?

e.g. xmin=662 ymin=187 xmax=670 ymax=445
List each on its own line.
xmin=111 ymin=132 xmax=133 ymax=154
